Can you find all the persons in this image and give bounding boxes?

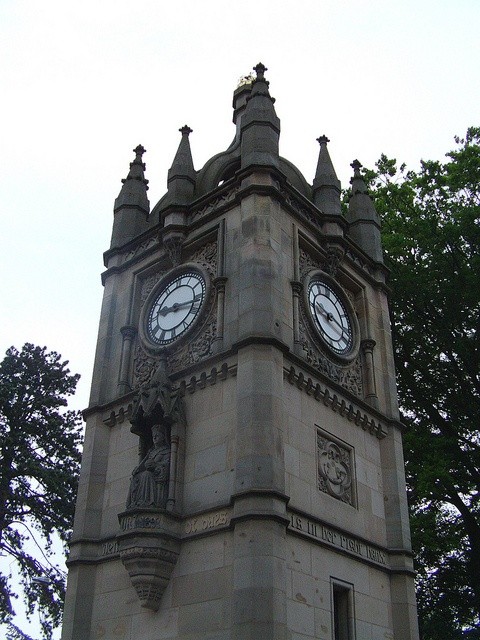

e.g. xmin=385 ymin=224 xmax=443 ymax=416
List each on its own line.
xmin=126 ymin=425 xmax=170 ymax=511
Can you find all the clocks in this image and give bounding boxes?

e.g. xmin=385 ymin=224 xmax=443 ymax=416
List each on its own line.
xmin=138 ymin=262 xmax=212 ymax=353
xmin=301 ymin=270 xmax=361 ymax=365
